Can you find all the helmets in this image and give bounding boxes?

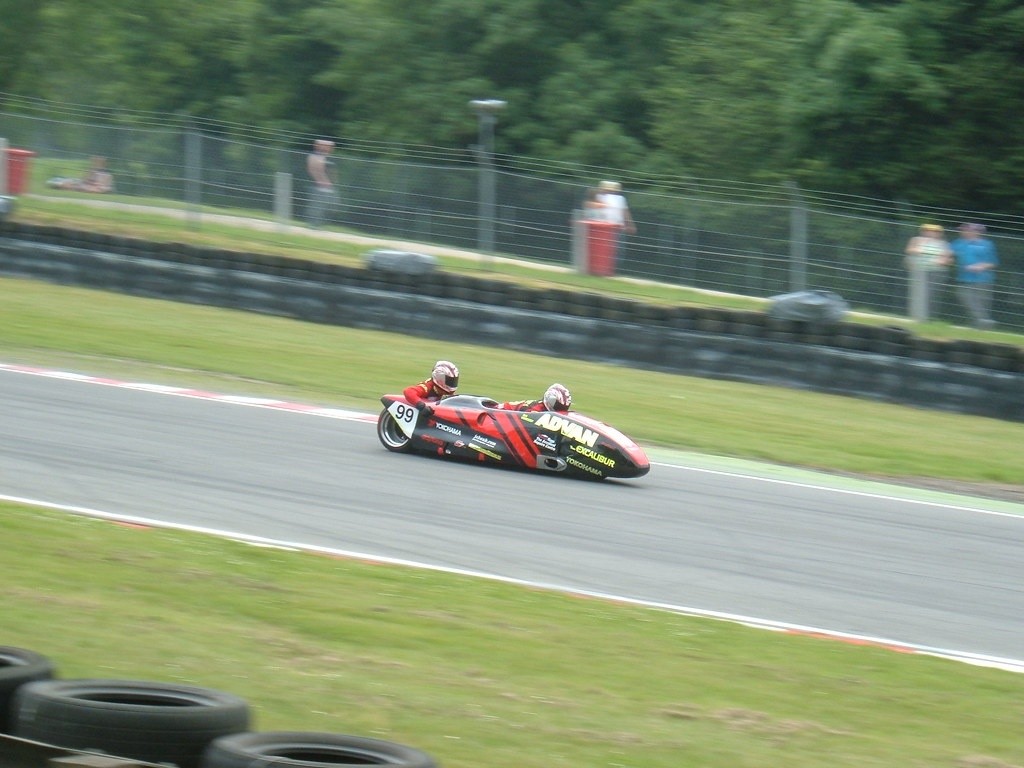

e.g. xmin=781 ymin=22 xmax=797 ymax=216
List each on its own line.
xmin=432 ymin=361 xmax=460 ymax=393
xmin=543 ymin=383 xmax=572 ymax=413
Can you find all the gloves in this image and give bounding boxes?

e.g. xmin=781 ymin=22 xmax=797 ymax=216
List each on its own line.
xmin=415 ymin=401 xmax=436 ymax=418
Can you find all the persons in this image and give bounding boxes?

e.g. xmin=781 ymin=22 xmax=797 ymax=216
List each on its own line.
xmin=586 ymin=181 xmax=636 ymax=271
xmin=82 ymin=156 xmax=111 ymax=194
xmin=305 ymin=140 xmax=335 ymax=226
xmin=904 ymin=222 xmax=951 ymax=319
xmin=492 ymin=382 xmax=571 ymax=413
xmin=404 ymin=361 xmax=459 ymax=418
xmin=947 ymin=224 xmax=1000 ymax=330
